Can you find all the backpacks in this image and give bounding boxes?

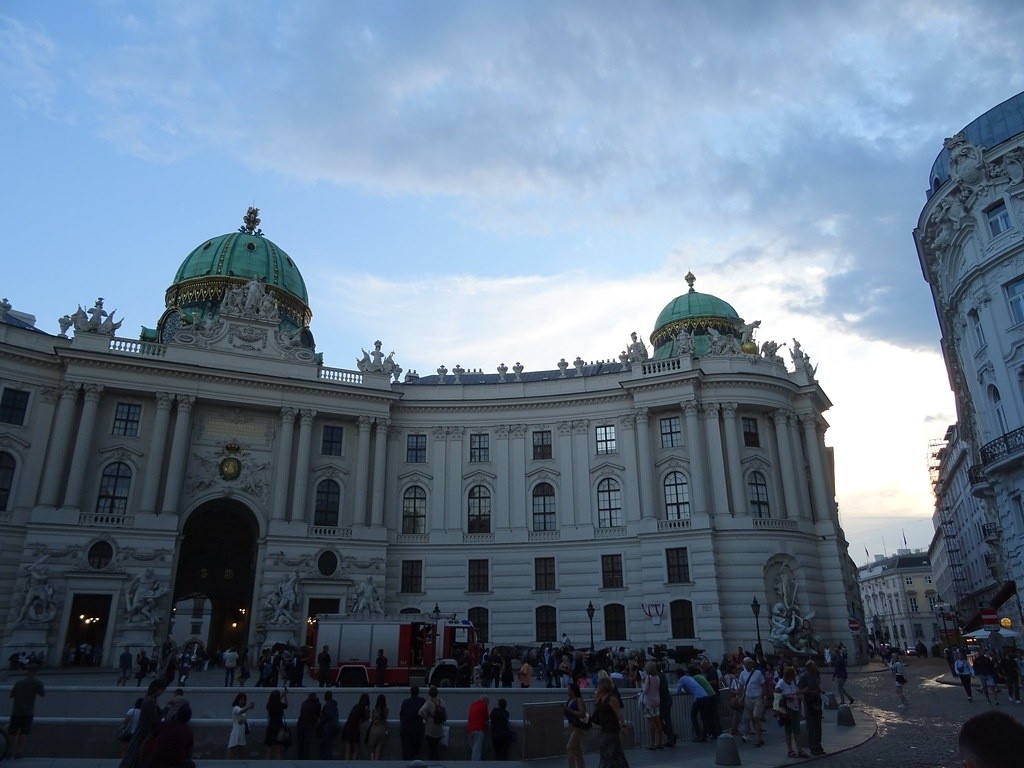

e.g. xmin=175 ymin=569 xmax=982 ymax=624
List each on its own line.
xmin=430 ymin=699 xmax=446 ymax=724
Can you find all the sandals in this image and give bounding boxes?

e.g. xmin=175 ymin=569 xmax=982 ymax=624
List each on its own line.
xmin=787 ymin=751 xmax=800 ymax=758
xmin=798 ymin=750 xmax=809 ymax=758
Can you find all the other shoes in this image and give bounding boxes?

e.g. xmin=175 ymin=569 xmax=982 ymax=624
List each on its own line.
xmin=646 ymin=744 xmax=656 ymax=750
xmin=656 ymin=744 xmax=663 ymax=749
xmin=967 ymin=695 xmax=1022 ymax=704
xmin=6 ymin=753 xmax=12 ymax=760
xmin=742 ymin=735 xmax=749 ymax=742
xmin=664 ymin=742 xmax=674 ymax=746
xmin=673 ymin=735 xmax=678 ymax=744
xmin=14 ymin=752 xmax=25 ymax=759
xmin=754 ymin=739 xmax=763 ymax=745
xmin=692 ymin=737 xmax=704 ymax=742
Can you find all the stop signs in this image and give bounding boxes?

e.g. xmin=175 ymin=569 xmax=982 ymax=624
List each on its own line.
xmin=981 ymin=609 xmax=1000 ymax=631
xmin=848 ymin=620 xmax=860 ymax=633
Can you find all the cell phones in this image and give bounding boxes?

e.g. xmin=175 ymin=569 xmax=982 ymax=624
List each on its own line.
xmin=284 ymin=686 xmax=287 ymax=691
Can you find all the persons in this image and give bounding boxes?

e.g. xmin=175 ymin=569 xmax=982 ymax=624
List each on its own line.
xmin=1 ymin=623 xmax=1023 ymax=768
xmin=16 ymin=556 xmax=54 ymax=624
xmin=124 ymin=567 xmax=167 ymax=623
xmin=371 ymin=340 xmax=384 ymax=369
xmin=771 ymin=560 xmax=805 ymax=645
xmin=266 ymin=571 xmax=304 ymax=623
xmin=351 ymin=575 xmax=384 ymax=613
xmin=624 ymin=327 xmax=817 ymax=379
xmin=220 ymin=274 xmax=280 ymax=320
xmin=87 ymin=298 xmax=108 ymax=325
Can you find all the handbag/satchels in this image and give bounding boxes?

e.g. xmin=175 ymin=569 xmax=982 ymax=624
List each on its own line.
xmin=592 ymin=694 xmax=616 ymax=725
xmin=439 ymin=726 xmax=450 ymax=747
xmin=730 ymin=697 xmax=741 ymax=710
xmin=244 ymin=722 xmax=250 ymax=735
xmin=509 ymin=730 xmax=517 ymax=743
xmin=895 ymin=674 xmax=904 ymax=682
xmin=117 ymin=723 xmax=130 ymax=741
xmin=316 ymin=724 xmax=323 ymax=737
xmin=278 ymin=726 xmax=289 ymax=742
xmin=572 ymin=711 xmax=593 ymax=729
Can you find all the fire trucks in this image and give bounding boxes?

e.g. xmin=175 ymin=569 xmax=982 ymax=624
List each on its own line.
xmin=308 ymin=613 xmax=484 ymax=688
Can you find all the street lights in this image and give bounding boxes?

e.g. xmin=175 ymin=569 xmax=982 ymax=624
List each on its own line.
xmin=751 ymin=600 xmax=761 ymax=644
xmin=948 ymin=604 xmax=957 ymax=631
xmin=586 ymin=600 xmax=596 ymax=651
xmin=937 ymin=594 xmax=951 ymax=655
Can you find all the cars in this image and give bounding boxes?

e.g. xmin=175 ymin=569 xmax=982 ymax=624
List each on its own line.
xmin=907 ymin=647 xmax=916 ymax=656
